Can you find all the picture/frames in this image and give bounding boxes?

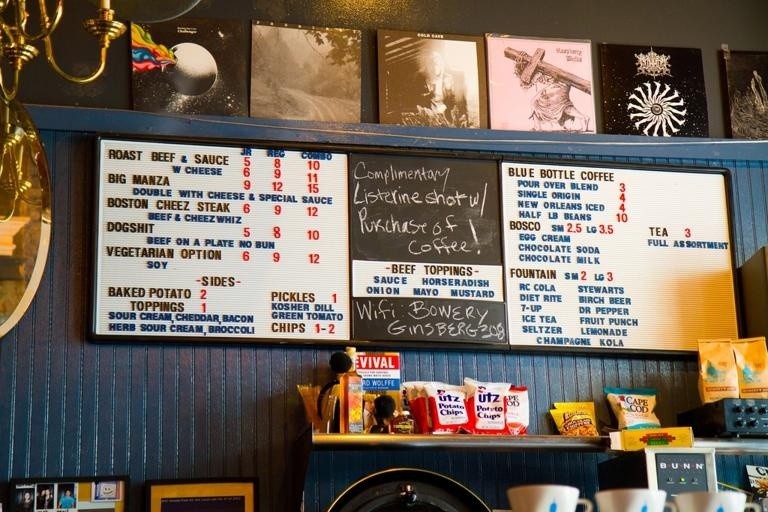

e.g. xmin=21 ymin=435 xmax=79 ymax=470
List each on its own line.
xmin=143 ymin=478 xmax=257 ymax=511
xmin=8 ymin=475 xmax=128 ymax=512
xmin=326 ymin=468 xmax=493 ymax=511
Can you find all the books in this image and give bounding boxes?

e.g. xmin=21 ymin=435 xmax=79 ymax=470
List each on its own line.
xmin=337 ymin=351 xmax=405 ymax=437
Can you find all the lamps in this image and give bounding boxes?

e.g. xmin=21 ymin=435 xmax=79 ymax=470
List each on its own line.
xmin=0 ymin=0 xmax=128 ymax=104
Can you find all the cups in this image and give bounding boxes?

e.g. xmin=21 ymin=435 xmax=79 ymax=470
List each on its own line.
xmin=673 ymin=490 xmax=760 ymax=512
xmin=506 ymin=483 xmax=593 ymax=512
xmin=594 ymin=488 xmax=678 ymax=512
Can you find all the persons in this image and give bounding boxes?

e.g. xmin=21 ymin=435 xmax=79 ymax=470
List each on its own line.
xmin=17 ymin=493 xmax=33 ymax=511
xmin=36 ymin=488 xmax=53 ymax=510
xmin=57 ymin=488 xmax=76 ymax=507
xmin=361 ymin=393 xmax=379 ymax=429
xmin=44 ymin=490 xmax=53 ymax=509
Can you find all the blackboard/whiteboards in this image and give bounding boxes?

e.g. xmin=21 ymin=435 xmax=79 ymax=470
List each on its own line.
xmin=86 ymin=133 xmax=355 ymax=342
xmin=350 ymin=144 xmax=510 ymax=355
xmin=497 ymin=150 xmax=746 ymax=362
